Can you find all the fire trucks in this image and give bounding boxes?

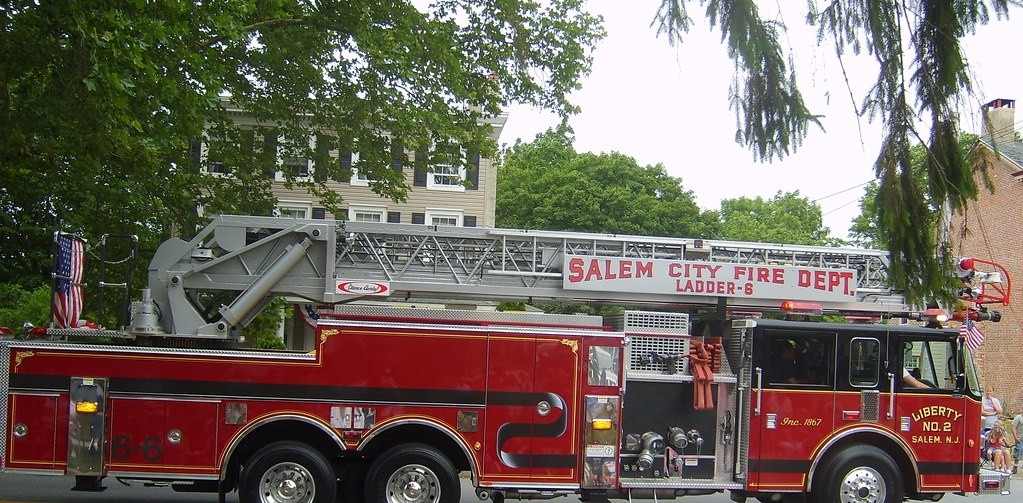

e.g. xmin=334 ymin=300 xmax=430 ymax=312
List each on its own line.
xmin=1 ymin=213 xmax=1011 ymax=503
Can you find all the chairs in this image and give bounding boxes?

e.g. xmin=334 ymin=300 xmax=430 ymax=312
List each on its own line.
xmin=982 ymin=427 xmax=996 ymax=466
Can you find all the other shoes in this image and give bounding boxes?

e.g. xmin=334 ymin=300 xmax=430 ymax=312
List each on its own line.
xmin=1006 ymin=470 xmax=1013 ymax=474
xmin=1000 ymin=468 xmax=1005 ymax=472
xmin=1012 ymin=466 xmax=1017 ymax=474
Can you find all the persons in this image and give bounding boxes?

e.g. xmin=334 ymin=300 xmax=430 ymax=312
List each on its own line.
xmin=980 ymin=385 xmax=1023 ymax=474
xmin=903 ymin=367 xmax=932 ymax=388
xmin=767 ymin=340 xmax=800 ymax=389
xmin=838 ymin=339 xmax=873 ymax=378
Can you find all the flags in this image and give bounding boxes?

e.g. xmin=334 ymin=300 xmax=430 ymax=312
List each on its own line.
xmin=51 ymin=231 xmax=84 ymax=329
xmin=960 ymin=317 xmax=985 ymax=354
